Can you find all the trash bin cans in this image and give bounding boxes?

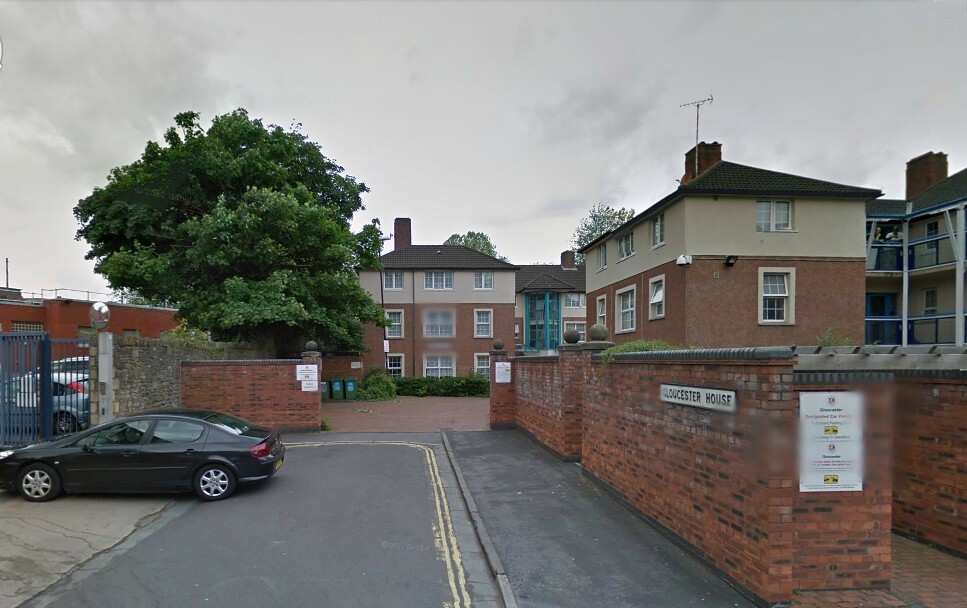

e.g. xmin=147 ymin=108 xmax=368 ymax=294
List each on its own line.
xmin=329 ymin=378 xmax=344 ymax=400
xmin=344 ymin=378 xmax=358 ymax=400
xmin=321 ymin=379 xmax=329 ymax=400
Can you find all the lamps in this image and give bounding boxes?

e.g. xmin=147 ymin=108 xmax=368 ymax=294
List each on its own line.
xmin=723 ymin=256 xmax=738 ymax=268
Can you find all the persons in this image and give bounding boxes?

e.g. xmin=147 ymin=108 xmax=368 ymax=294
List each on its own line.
xmin=866 ymin=227 xmax=883 ymax=241
xmin=886 ymin=226 xmax=904 ymax=240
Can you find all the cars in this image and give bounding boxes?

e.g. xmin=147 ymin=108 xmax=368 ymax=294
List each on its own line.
xmin=0 ymin=408 xmax=285 ymax=501
xmin=4 ymin=356 xmax=90 ymax=435
